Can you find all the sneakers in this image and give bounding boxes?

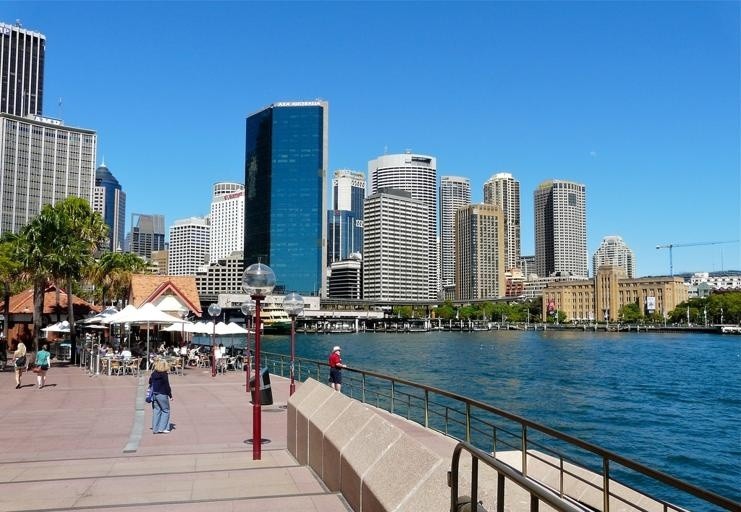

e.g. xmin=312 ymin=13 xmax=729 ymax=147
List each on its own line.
xmin=158 ymin=429 xmax=170 ymax=434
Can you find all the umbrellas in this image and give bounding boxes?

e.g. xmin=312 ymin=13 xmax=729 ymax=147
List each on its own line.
xmin=40 ymin=301 xmax=255 ymax=335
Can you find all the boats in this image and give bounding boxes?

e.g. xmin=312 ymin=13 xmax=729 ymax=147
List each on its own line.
xmin=253 ymin=296 xmax=299 ymax=334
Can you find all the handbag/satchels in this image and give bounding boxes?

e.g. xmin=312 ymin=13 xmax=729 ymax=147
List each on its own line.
xmin=16 ymin=356 xmax=27 ymax=367
xmin=32 ymin=365 xmax=41 ymax=375
xmin=145 ymin=386 xmax=153 ymax=404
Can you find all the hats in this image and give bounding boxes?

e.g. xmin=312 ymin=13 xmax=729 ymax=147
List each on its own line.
xmin=332 ymin=346 xmax=342 ymax=353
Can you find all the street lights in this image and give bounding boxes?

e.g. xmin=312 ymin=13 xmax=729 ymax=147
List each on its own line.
xmin=206 ymin=302 xmax=223 ymax=377
xmin=238 ymin=260 xmax=278 ymax=459
xmin=176 ymin=306 xmax=189 ymax=345
xmin=279 ymin=290 xmax=305 ymax=398
xmin=240 ymin=297 xmax=256 ymax=392
xmin=527 ymin=299 xmax=532 ymax=326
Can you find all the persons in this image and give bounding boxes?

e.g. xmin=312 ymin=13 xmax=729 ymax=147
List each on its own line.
xmin=76 ymin=331 xmax=252 ymax=372
xmin=329 ymin=346 xmax=348 ymax=392
xmin=148 ymin=362 xmax=173 ymax=433
xmin=12 ymin=343 xmax=29 ymax=389
xmin=35 ymin=344 xmax=51 ymax=389
xmin=0 ymin=335 xmax=11 ymax=371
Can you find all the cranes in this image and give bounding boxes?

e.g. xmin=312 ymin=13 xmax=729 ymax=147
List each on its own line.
xmin=706 ymin=239 xmax=740 ymax=273
xmin=653 ymin=241 xmax=712 ymax=277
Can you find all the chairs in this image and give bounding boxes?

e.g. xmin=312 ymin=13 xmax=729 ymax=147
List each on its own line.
xmin=149 ymin=346 xmax=248 ymax=374
xmin=89 ymin=339 xmax=143 ymax=377
